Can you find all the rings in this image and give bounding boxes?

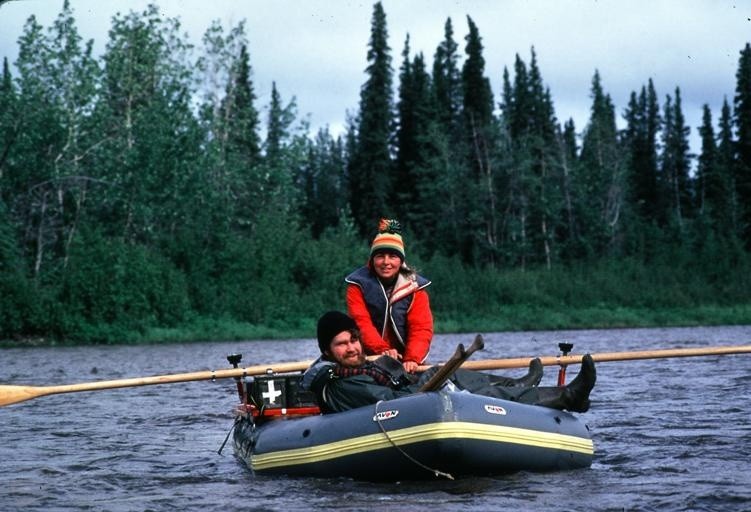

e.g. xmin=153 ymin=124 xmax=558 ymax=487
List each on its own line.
xmin=389 ymin=354 xmax=395 ymax=357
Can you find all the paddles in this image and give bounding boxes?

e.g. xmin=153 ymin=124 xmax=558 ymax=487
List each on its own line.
xmin=0 ymin=354 xmax=382 ymax=406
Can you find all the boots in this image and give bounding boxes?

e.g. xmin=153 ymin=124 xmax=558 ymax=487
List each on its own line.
xmin=536 ymin=353 xmax=596 ymax=413
xmin=489 ymin=357 xmax=542 ymax=388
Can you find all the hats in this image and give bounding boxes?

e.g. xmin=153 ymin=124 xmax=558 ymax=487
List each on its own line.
xmin=317 ymin=312 xmax=360 ymax=357
xmin=367 ymin=219 xmax=405 ymax=270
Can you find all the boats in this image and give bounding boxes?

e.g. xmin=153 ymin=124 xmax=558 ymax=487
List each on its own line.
xmin=226 ymin=340 xmax=594 ymax=483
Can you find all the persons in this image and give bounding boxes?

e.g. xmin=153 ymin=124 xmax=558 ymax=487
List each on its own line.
xmin=300 ymin=310 xmax=599 ymax=415
xmin=344 ymin=219 xmax=436 ymax=373
